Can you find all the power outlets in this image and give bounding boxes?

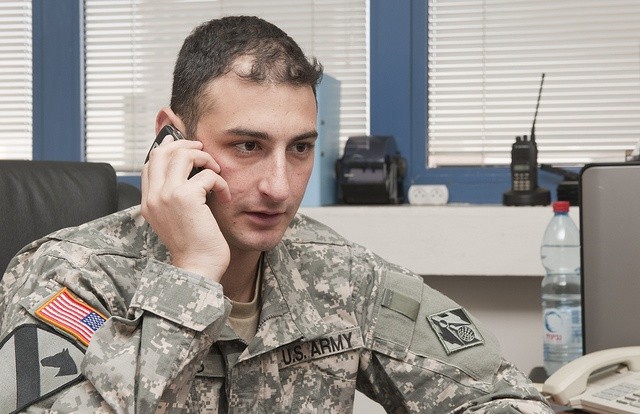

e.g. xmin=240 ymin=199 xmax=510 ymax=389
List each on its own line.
xmin=409 ymin=181 xmax=450 ymax=207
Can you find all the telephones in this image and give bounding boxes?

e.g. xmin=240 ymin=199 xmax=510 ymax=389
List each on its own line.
xmin=503 ymin=73 xmax=551 ymax=207
xmin=540 ymin=346 xmax=640 ymax=414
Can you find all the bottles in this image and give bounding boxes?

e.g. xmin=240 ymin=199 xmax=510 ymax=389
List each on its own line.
xmin=540 ymin=199 xmax=585 ymax=377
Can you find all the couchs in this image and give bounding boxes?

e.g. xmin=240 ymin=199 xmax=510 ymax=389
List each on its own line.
xmin=1 ymin=163 xmax=141 ymax=286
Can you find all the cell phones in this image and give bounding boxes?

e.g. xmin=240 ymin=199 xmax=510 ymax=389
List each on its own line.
xmin=143 ymin=123 xmax=213 ymax=204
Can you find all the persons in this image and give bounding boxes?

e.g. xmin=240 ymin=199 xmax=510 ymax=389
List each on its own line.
xmin=1 ymin=15 xmax=557 ymax=414
